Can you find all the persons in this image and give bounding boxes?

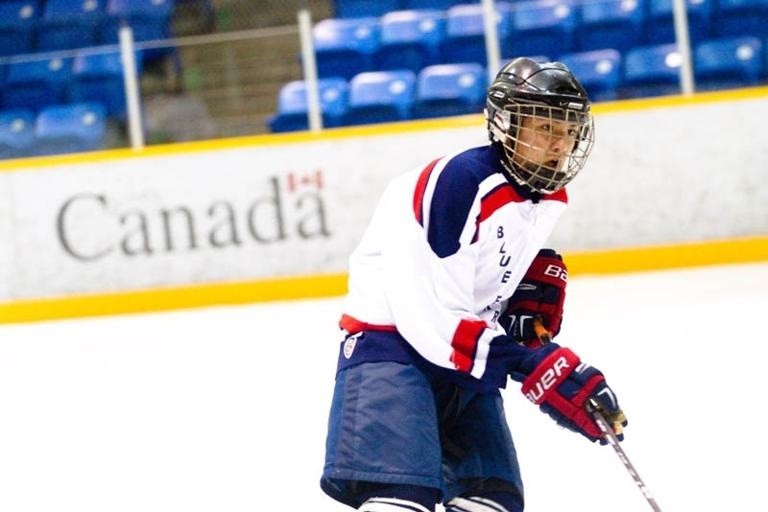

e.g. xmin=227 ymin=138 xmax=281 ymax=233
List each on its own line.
xmin=318 ymin=57 xmax=629 ymax=512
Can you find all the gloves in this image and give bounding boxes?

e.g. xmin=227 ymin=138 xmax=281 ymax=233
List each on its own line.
xmin=513 ymin=342 xmax=626 ymax=444
xmin=499 ymin=249 xmax=568 ymax=347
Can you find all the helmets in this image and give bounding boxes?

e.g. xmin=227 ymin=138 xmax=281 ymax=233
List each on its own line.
xmin=488 ymin=57 xmax=594 ymax=202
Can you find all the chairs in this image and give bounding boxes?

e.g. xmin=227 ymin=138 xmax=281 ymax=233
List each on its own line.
xmin=267 ymin=0 xmax=768 ymax=133
xmin=1 ymin=0 xmax=216 ymax=155
